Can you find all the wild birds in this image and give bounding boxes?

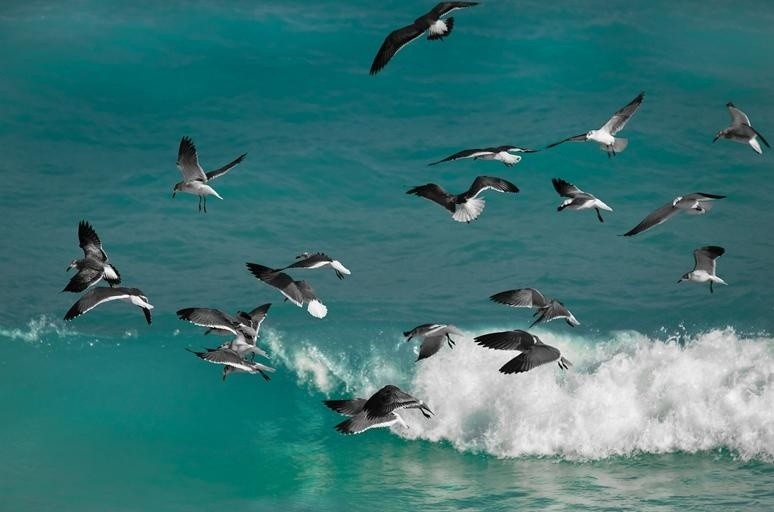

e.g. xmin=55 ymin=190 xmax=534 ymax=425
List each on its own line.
xmin=320 ymin=384 xmax=436 ymax=436
xmin=402 ymin=322 xmax=465 ymax=363
xmin=171 ymin=135 xmax=248 ymax=214
xmin=473 ymin=329 xmax=574 ymax=375
xmin=404 ymin=174 xmax=520 ymax=224
xmin=244 ymin=261 xmax=328 ymax=320
xmin=58 ymin=219 xmax=122 ymax=294
xmin=550 ymin=177 xmax=614 ymax=223
xmin=619 ymin=191 xmax=728 ymax=238
xmin=676 ymin=245 xmax=729 ymax=294
xmin=427 ymin=144 xmax=537 ymax=168
xmin=712 ymin=101 xmax=771 ymax=155
xmin=269 ymin=250 xmax=352 ymax=281
xmin=544 ymin=89 xmax=645 ymax=159
xmin=488 ymin=286 xmax=582 ymax=329
xmin=175 ymin=302 xmax=272 ymax=383
xmin=367 ymin=0 xmax=479 ymax=76
xmin=63 ymin=286 xmax=156 ymax=328
